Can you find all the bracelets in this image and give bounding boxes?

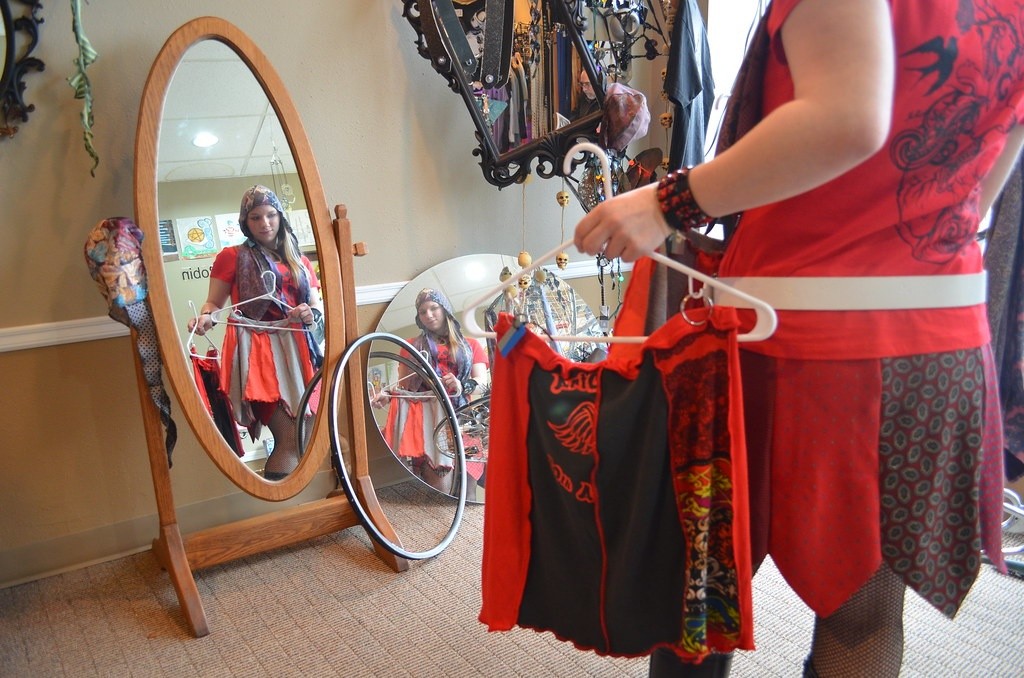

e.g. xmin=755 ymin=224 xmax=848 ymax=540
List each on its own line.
xmin=203 ymin=311 xmax=217 ymax=326
xmin=657 ymin=165 xmax=712 ymax=233
xmin=384 ymin=390 xmax=392 ymax=402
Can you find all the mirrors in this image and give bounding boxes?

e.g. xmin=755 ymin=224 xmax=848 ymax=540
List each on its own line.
xmin=401 ymin=0 xmax=608 ymax=191
xmin=84 ymin=15 xmax=410 ymax=637
xmin=367 ymin=253 xmax=607 ymax=506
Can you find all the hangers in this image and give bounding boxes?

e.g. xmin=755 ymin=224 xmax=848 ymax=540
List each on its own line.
xmin=368 ymin=382 xmax=389 ymax=426
xmin=381 ymin=350 xmax=461 ymax=398
xmin=210 ymin=271 xmax=317 ymax=332
xmin=461 ymin=142 xmax=777 ymax=342
xmin=186 ymin=300 xmax=222 ymax=359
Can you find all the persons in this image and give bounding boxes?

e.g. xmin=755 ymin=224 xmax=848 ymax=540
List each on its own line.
xmin=188 ymin=185 xmax=325 ymax=481
xmin=574 ymin=1 xmax=1023 ymax=678
xmin=554 ymin=53 xmax=604 ymax=132
xmin=370 ymin=287 xmax=488 ymax=502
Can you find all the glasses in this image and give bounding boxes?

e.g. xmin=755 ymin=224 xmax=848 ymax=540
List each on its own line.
xmin=578 ymin=82 xmax=591 ymax=88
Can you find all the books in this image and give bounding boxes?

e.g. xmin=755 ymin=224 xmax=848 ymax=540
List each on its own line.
xmin=552 ymin=111 xmax=572 ymax=130
